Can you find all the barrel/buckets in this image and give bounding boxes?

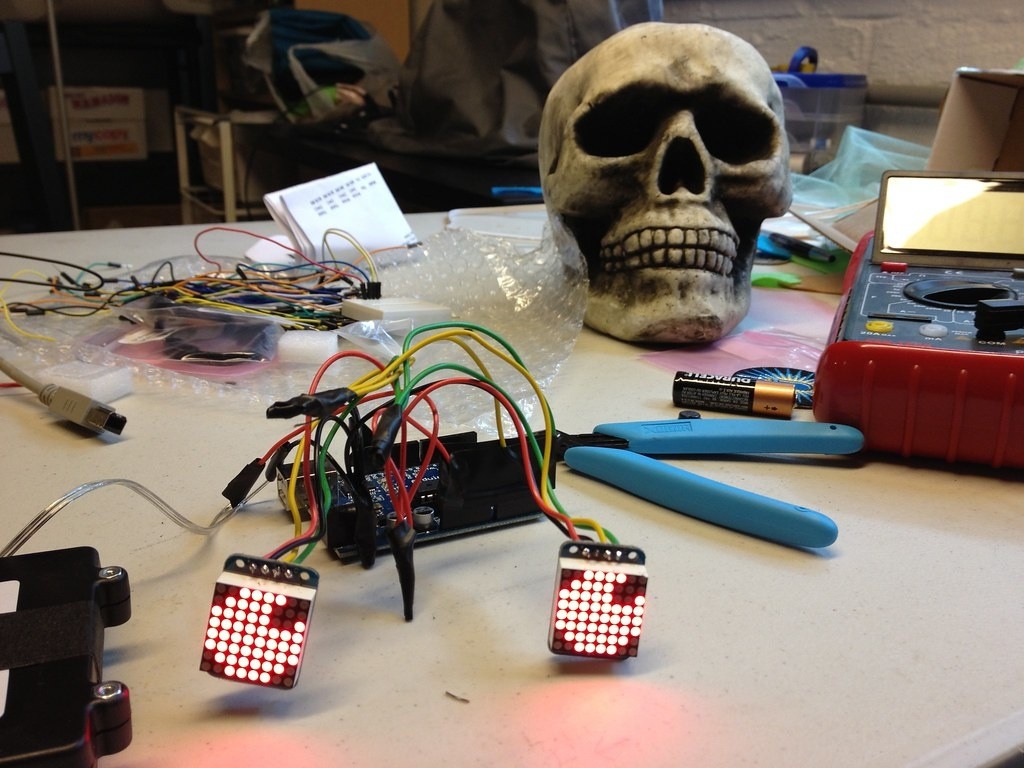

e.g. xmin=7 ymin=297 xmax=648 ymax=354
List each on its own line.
xmin=770 ymin=46 xmax=870 ymax=173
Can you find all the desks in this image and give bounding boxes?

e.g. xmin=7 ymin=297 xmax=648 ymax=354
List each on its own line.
xmin=0 ymin=187 xmax=1024 ymax=768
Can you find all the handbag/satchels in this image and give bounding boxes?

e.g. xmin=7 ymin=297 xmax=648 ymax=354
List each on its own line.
xmin=246 ymin=12 xmax=400 ymax=127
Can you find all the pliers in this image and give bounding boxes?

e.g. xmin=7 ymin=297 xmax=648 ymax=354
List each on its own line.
xmin=526 ymin=419 xmax=864 ymax=548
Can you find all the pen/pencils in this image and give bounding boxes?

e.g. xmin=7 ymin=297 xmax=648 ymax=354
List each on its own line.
xmin=760 ymin=227 xmax=835 ymax=263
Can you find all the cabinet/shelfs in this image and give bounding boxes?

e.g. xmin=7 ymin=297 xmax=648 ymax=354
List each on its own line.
xmin=173 ymin=101 xmax=330 ymax=224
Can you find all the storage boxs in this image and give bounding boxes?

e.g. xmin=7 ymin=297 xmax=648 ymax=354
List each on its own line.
xmin=922 ymin=65 xmax=1024 ymax=172
xmin=48 ymin=84 xmax=173 ymax=161
xmin=766 ymin=46 xmax=870 ymax=172
